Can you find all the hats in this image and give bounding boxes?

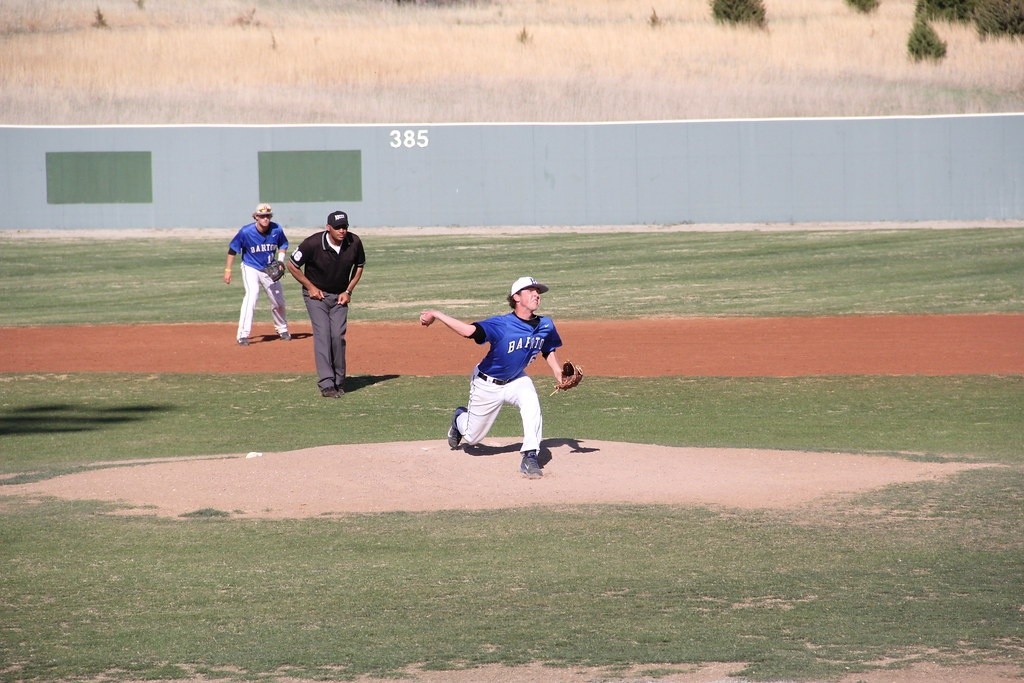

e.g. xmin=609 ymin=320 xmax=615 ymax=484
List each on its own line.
xmin=510 ymin=277 xmax=549 ymax=298
xmin=328 ymin=211 xmax=348 ymax=227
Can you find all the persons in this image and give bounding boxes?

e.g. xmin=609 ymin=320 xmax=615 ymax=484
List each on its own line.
xmin=419 ymin=276 xmax=584 ymax=480
xmin=286 ymin=210 xmax=367 ymax=398
xmin=223 ymin=203 xmax=291 ymax=346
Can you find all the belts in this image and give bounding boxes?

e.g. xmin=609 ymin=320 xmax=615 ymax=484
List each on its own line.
xmin=477 ymin=372 xmax=518 ymax=385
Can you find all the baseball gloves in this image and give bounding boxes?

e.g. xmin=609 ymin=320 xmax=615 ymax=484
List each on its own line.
xmin=555 ymin=360 xmax=584 ymax=388
xmin=264 ymin=260 xmax=285 ymax=281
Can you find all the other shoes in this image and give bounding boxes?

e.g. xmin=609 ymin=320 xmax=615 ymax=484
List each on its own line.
xmin=321 ymin=386 xmax=338 ymax=398
xmin=334 ymin=387 xmax=344 ymax=396
xmin=238 ymin=337 xmax=250 ymax=345
xmin=278 ymin=331 xmax=292 ymax=340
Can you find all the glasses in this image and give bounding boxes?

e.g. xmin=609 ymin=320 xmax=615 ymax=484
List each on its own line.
xmin=331 ymin=224 xmax=348 ymax=230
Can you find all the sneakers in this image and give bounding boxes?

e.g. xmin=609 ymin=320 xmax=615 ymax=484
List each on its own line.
xmin=520 ymin=451 xmax=542 ymax=479
xmin=447 ymin=407 xmax=469 ymax=451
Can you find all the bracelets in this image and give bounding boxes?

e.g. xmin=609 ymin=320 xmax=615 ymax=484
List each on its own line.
xmin=225 ymin=269 xmax=231 ymax=271
xmin=346 ymin=290 xmax=352 ymax=295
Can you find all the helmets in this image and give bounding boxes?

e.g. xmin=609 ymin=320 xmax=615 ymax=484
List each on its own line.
xmin=252 ymin=203 xmax=273 ymax=217
